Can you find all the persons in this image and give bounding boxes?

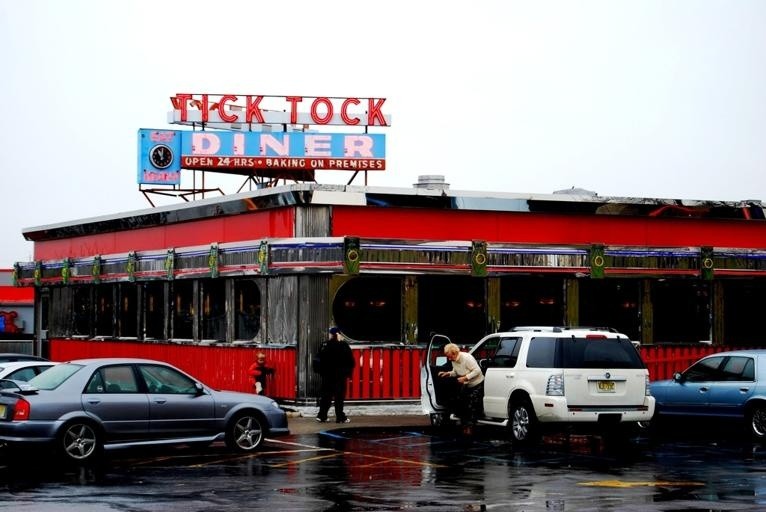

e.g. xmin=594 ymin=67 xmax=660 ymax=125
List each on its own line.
xmin=311 ymin=326 xmax=355 ymax=424
xmin=247 ymin=352 xmax=274 ymax=395
xmin=438 ymin=341 xmax=486 ymax=436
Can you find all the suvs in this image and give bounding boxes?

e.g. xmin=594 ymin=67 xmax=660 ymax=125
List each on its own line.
xmin=420 ymin=326 xmax=656 ymax=445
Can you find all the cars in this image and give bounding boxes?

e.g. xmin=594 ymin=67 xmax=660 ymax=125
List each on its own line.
xmin=635 ymin=349 xmax=766 ymax=440
xmin=0 ymin=358 xmax=290 ymax=468
xmin=0 ymin=361 xmax=111 ymax=391
xmin=0 ymin=353 xmax=52 ymax=363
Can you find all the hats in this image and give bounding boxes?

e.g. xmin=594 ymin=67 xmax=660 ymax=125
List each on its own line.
xmin=328 ymin=326 xmax=341 ymax=334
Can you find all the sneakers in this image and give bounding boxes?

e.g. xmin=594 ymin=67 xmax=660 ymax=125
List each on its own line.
xmin=336 ymin=418 xmax=352 ymax=424
xmin=315 ymin=416 xmax=331 ymax=423
